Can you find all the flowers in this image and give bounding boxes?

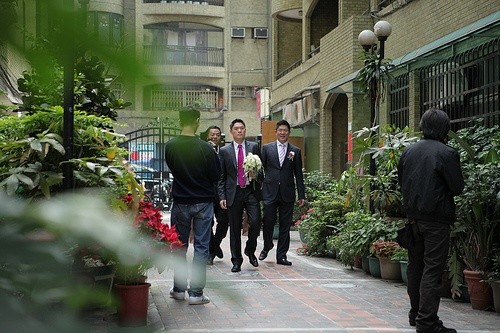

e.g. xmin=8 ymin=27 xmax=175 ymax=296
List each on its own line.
xmin=241 ymin=151 xmax=266 ymax=181
xmin=293 ymin=186 xmax=327 ymax=256
xmin=288 ymin=151 xmax=296 ymax=162
xmin=120 ymin=194 xmax=188 ymax=286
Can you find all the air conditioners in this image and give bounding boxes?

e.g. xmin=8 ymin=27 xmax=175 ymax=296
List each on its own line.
xmin=252 ymin=87 xmax=264 ymax=97
xmin=231 ymin=27 xmax=245 ymax=39
xmin=252 ymin=28 xmax=268 ymax=39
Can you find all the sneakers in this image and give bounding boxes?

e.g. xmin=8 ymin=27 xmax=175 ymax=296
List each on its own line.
xmin=170 ymin=286 xmax=185 ymax=300
xmin=189 ymin=291 xmax=210 ymax=304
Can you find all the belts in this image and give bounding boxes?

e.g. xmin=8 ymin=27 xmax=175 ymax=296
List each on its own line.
xmin=236 ymin=185 xmax=252 ymax=189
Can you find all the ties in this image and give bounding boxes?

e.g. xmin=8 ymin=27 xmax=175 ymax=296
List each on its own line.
xmin=238 ymin=145 xmax=246 ymax=186
xmin=279 ymin=144 xmax=286 ymax=166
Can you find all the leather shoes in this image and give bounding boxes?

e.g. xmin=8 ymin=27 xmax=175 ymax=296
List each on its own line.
xmin=214 ymin=238 xmax=223 ymax=258
xmin=259 ymin=244 xmax=274 ymax=260
xmin=277 ymin=258 xmax=292 ymax=266
xmin=206 ymin=253 xmax=214 ymax=265
xmin=244 ymin=250 xmax=259 ymax=267
xmin=231 ymin=259 xmax=243 ymax=272
xmin=409 ymin=312 xmax=417 ymax=326
xmin=417 ymin=325 xmax=457 ymax=333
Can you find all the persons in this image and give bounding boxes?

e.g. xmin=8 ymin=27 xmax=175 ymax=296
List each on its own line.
xmin=217 ymin=119 xmax=263 ymax=272
xmin=397 ymin=109 xmax=465 ymax=333
xmin=164 ymin=106 xmax=221 ymax=303
xmin=259 ymin=120 xmax=305 ymax=265
xmin=205 ymin=126 xmax=230 ymax=265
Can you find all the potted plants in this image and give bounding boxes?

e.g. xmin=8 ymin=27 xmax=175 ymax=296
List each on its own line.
xmin=79 ymin=254 xmax=117 ymax=313
xmin=324 ymin=117 xmax=500 ymax=314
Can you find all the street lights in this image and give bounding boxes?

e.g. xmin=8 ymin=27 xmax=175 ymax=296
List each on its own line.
xmin=358 ymin=20 xmax=392 ymax=214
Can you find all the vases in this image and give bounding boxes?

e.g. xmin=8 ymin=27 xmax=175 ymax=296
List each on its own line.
xmin=113 ymin=283 xmax=151 ymax=328
xmin=298 ymin=228 xmax=309 ymax=243
xmin=272 ymin=225 xmax=280 ymax=240
